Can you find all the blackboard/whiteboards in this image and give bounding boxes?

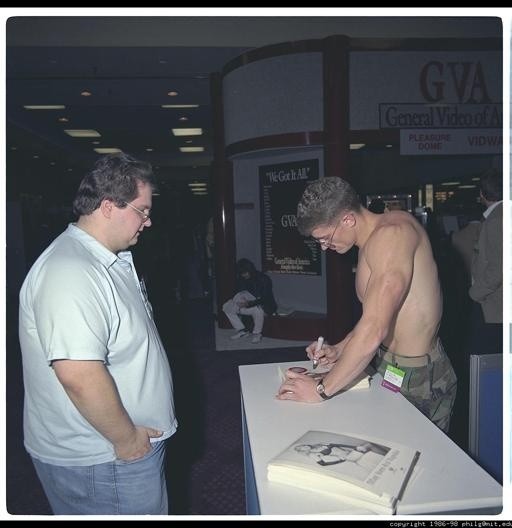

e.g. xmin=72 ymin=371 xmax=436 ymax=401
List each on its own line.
xmin=258 ymin=158 xmax=322 ymax=276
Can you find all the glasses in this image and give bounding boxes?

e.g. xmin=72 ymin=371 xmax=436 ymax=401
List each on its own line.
xmin=314 ymin=219 xmax=342 ymax=249
xmin=123 ymin=200 xmax=153 ymax=222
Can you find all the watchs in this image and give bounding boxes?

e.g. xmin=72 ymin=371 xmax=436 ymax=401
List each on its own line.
xmin=315 ymin=374 xmax=329 ymax=404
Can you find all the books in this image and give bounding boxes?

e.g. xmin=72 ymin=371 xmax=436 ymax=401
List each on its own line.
xmin=267 ymin=472 xmax=397 ymax=515
xmin=268 ymin=430 xmax=418 ymax=509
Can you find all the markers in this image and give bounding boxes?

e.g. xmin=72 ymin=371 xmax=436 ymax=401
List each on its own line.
xmin=313 ymin=336 xmax=324 ymax=370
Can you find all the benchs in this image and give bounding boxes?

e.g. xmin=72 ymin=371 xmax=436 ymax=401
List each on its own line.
xmin=216 ymin=278 xmax=332 ymax=345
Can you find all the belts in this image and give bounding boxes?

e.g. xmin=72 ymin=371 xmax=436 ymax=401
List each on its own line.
xmin=375 ymin=339 xmax=445 ymax=370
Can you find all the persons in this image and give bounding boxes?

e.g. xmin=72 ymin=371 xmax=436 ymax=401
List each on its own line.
xmin=18 ymin=147 xmax=182 ymax=514
xmin=274 ymin=169 xmax=457 ymax=435
xmin=221 ymin=257 xmax=281 ymax=344
xmin=295 ymin=442 xmax=385 ymax=476
xmin=434 ymin=168 xmax=503 ymax=354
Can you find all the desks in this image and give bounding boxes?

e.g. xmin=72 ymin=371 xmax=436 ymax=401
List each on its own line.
xmin=237 ymin=324 xmax=503 ymax=517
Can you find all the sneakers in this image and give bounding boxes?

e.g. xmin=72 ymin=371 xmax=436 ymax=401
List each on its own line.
xmin=229 ymin=329 xmax=251 ymax=341
xmin=250 ymin=333 xmax=263 ymax=344
